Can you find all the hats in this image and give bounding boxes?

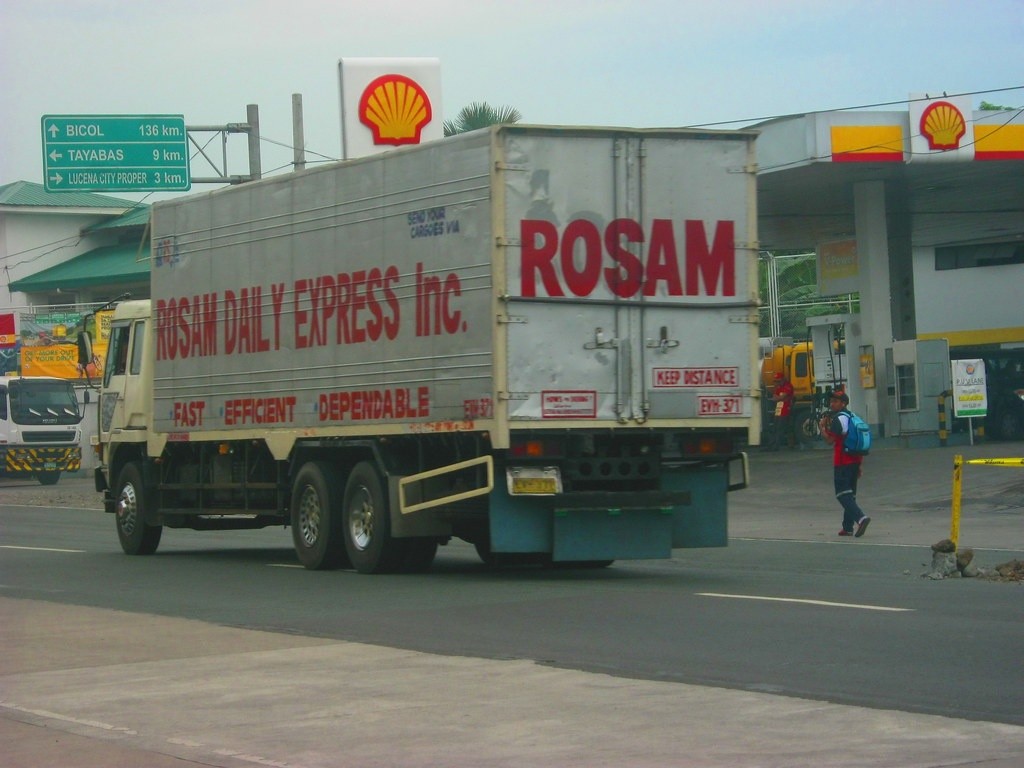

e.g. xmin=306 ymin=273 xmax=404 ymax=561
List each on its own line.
xmin=774 ymin=371 xmax=784 ymax=379
xmin=829 ymin=391 xmax=849 ymax=404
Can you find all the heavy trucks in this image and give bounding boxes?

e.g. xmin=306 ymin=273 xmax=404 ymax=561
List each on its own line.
xmin=79 ymin=121 xmax=761 ymax=573
xmin=759 ymin=335 xmax=847 ymax=443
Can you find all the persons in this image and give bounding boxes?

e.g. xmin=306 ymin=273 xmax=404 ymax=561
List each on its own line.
xmin=762 ymin=372 xmax=793 ymax=451
xmin=819 ymin=391 xmax=871 ymax=537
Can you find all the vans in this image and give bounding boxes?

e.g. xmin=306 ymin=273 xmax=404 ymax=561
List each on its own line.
xmin=949 ymin=348 xmax=1024 ymax=441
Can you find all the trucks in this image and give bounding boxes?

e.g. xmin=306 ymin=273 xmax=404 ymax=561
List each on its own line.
xmin=1 ymin=371 xmax=89 ymax=486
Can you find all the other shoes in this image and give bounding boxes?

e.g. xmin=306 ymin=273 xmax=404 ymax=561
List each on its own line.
xmin=854 ymin=517 xmax=871 ymax=537
xmin=838 ymin=529 xmax=853 ymax=536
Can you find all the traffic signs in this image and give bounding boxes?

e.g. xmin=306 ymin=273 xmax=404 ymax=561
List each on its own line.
xmin=42 ymin=115 xmax=190 ymax=192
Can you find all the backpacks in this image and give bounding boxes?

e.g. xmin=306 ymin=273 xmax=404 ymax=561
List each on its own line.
xmin=833 ymin=412 xmax=871 ymax=456
